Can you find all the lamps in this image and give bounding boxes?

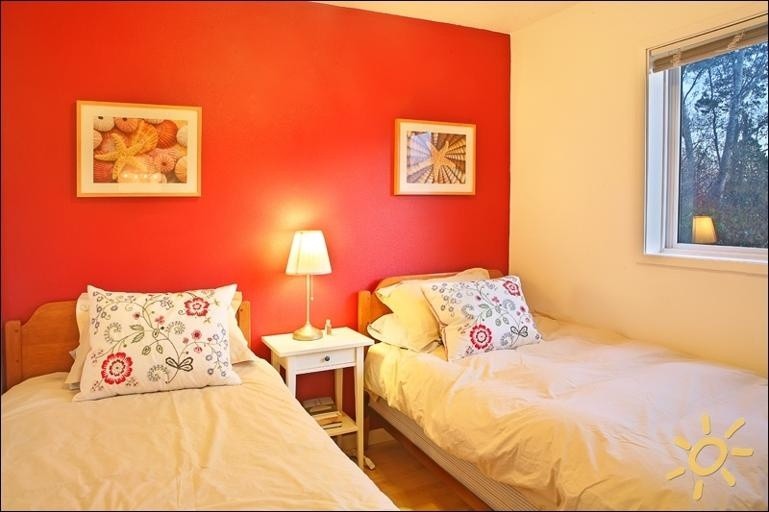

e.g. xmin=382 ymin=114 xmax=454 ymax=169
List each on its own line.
xmin=693 ymin=215 xmax=718 ymax=243
xmin=285 ymin=229 xmax=332 ymax=342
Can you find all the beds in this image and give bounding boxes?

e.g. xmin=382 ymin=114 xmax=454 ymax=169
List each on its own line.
xmin=357 ymin=269 xmax=767 ymax=512
xmin=2 ymin=298 xmax=400 ymax=512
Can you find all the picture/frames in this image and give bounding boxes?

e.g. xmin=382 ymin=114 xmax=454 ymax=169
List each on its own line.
xmin=394 ymin=117 xmax=475 ymax=197
xmin=75 ymin=98 xmax=202 ymax=198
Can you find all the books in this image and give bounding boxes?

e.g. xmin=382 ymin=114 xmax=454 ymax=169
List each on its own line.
xmin=302 ymin=397 xmax=343 ymax=429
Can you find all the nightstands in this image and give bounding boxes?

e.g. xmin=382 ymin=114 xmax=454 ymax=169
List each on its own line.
xmin=259 ymin=326 xmax=375 ymax=472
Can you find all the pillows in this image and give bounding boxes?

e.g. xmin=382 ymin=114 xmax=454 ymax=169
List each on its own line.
xmin=365 ymin=265 xmax=542 ymax=362
xmin=68 ymin=282 xmax=260 ymax=404
